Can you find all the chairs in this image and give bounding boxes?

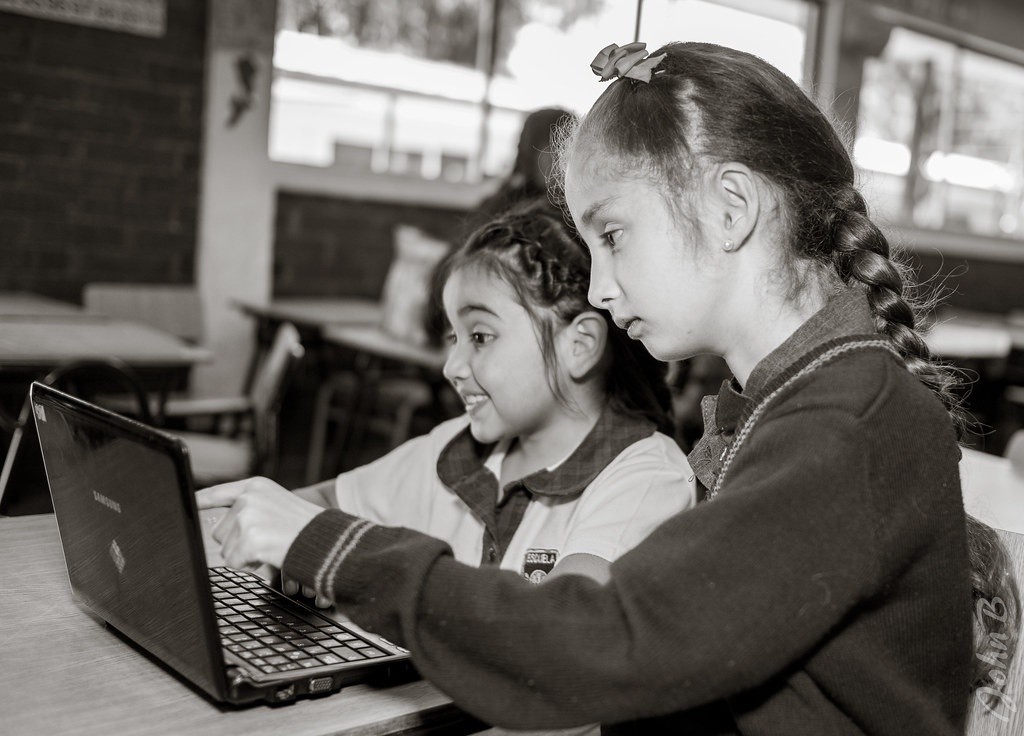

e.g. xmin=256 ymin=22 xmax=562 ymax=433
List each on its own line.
xmin=160 ymin=320 xmax=305 ymax=485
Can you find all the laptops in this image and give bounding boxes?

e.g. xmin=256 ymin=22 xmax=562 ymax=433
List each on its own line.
xmin=31 ymin=380 xmax=424 ymax=705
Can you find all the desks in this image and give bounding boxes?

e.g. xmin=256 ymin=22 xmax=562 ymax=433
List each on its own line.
xmin=0 ymin=508 xmax=495 ymax=736
xmin=0 ymin=292 xmax=211 ymax=516
xmin=234 ymin=297 xmax=449 ymax=461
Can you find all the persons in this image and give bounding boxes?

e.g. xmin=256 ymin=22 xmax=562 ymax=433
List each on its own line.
xmin=194 ymin=41 xmax=1024 ymax=736
xmin=425 ymin=108 xmax=579 ymax=353
xmin=280 ymin=195 xmax=697 ymax=611
